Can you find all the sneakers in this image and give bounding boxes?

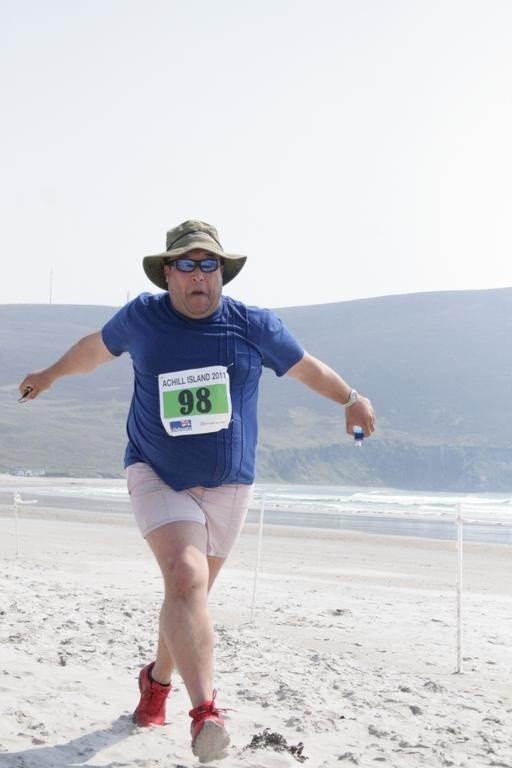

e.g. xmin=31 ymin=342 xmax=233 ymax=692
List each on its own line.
xmin=133 ymin=661 xmax=173 ymax=727
xmin=188 ymin=701 xmax=231 ymax=763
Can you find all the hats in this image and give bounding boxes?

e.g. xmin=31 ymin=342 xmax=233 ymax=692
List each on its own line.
xmin=142 ymin=219 xmax=247 ymax=291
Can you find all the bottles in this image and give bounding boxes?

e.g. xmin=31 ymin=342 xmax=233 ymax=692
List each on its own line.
xmin=353 ymin=426 xmax=364 ymax=447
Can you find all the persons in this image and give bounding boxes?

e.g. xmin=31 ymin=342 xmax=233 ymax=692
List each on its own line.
xmin=16 ymin=219 xmax=378 ymax=763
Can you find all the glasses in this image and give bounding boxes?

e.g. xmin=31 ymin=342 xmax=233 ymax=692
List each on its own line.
xmin=167 ymin=258 xmax=222 ymax=272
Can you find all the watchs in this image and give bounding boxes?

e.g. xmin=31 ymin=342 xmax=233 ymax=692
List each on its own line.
xmin=342 ymin=388 xmax=359 ymax=409
